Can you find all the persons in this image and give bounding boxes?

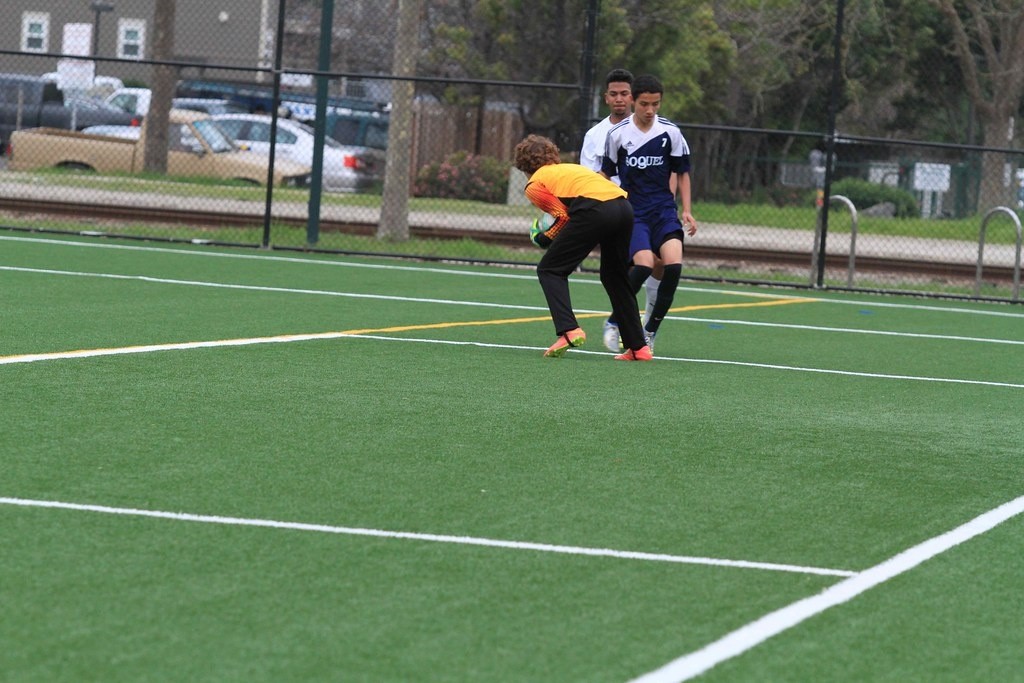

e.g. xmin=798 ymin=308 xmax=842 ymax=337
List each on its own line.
xmin=599 ymin=73 xmax=697 ymax=350
xmin=512 ymin=134 xmax=652 ymax=360
xmin=580 ymin=69 xmax=663 ymax=328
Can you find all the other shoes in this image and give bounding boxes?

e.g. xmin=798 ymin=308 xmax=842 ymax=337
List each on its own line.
xmin=619 ymin=314 xmax=644 ymax=348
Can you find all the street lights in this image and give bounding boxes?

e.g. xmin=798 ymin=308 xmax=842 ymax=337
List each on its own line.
xmin=89 ymin=0 xmax=114 ymax=76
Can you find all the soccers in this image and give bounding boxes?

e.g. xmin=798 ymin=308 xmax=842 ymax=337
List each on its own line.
xmin=542 ymin=212 xmax=556 ymax=231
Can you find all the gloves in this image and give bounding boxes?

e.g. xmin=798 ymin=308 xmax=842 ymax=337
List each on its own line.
xmin=529 ymin=218 xmax=540 ymax=247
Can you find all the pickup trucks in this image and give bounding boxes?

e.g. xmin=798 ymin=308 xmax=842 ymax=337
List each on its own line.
xmin=7 ymin=107 xmax=312 ymax=190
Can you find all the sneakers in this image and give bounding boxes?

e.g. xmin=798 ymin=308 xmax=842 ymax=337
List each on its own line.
xmin=603 ymin=319 xmax=620 ymax=353
xmin=613 ymin=345 xmax=653 ymax=360
xmin=543 ymin=327 xmax=586 ymax=358
xmin=642 ymin=328 xmax=656 ymax=355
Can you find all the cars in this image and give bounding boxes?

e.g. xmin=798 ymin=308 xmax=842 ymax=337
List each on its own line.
xmin=81 ymin=115 xmax=380 ymax=193
xmin=0 ymin=72 xmax=390 ymax=155
